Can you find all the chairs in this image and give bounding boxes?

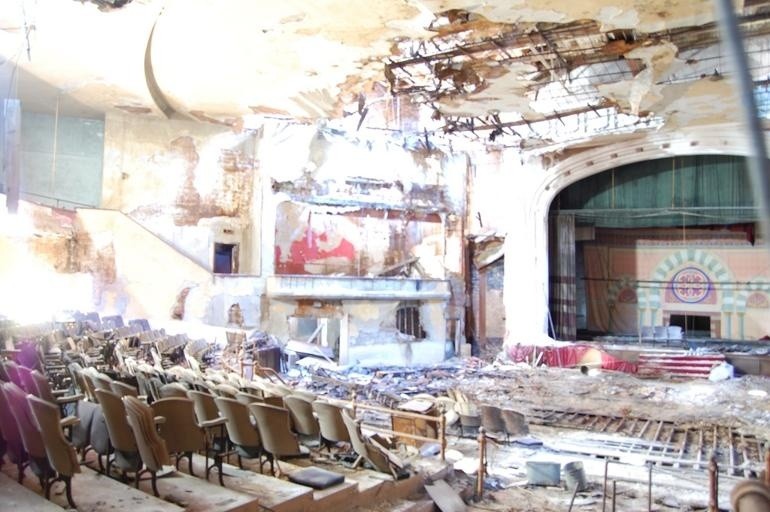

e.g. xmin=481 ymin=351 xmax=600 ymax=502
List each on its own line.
xmin=640 ymin=325 xmax=684 ymax=349
xmin=0 ymin=312 xmax=531 ymax=510
